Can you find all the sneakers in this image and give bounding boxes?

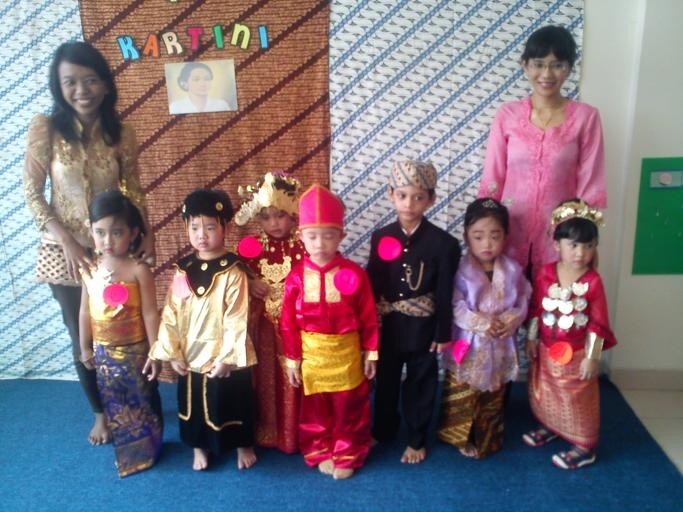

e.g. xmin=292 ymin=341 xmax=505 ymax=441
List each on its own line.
xmin=523 ymin=426 xmax=559 ymax=446
xmin=552 ymin=446 xmax=596 ymax=470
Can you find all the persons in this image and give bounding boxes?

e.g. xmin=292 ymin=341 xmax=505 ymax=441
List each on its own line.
xmin=437 ymin=196 xmax=533 ymax=460
xmin=365 ymin=161 xmax=462 ymax=465
xmin=521 ymin=198 xmax=618 ymax=471
xmin=477 ymin=26 xmax=608 ymax=330
xmin=169 ymin=62 xmax=230 ymax=117
xmin=281 ymin=183 xmax=380 ymax=481
xmin=171 ymin=230 xmax=256 ymax=472
xmin=22 ymin=41 xmax=157 ymax=446
xmin=235 ymin=172 xmax=310 ymax=455
xmin=76 ymin=190 xmax=163 ymax=478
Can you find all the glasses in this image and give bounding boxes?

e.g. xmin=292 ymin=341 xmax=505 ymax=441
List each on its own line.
xmin=526 ymin=62 xmax=573 ymax=74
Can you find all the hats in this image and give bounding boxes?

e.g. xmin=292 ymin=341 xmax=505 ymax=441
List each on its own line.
xmin=295 ymin=184 xmax=347 ymax=232
xmin=387 ymin=158 xmax=437 ymax=190
xmin=183 ymin=188 xmax=232 ymax=222
